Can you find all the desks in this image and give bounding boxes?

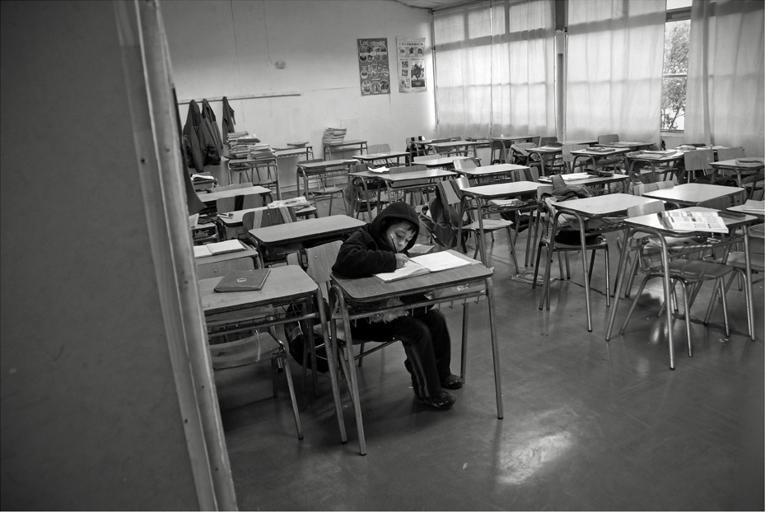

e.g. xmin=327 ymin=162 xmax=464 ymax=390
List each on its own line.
xmin=186 ymin=181 xmax=505 ymax=456
xmin=216 ymin=136 xmax=757 ymax=369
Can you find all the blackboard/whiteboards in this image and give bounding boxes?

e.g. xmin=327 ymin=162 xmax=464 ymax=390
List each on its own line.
xmin=161 ymin=1 xmax=431 ymax=104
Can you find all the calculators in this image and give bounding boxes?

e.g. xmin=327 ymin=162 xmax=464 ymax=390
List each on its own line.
xmin=584 ymin=168 xmax=613 ymax=177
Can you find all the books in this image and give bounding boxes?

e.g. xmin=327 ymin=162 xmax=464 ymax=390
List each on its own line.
xmin=658 ymin=207 xmax=729 ymax=235
xmin=269 ymin=196 xmax=310 ymax=212
xmin=561 ymin=172 xmax=598 ymax=180
xmin=322 ymin=128 xmax=346 ymax=144
xmin=223 ymin=130 xmax=276 ymax=163
xmin=586 ymin=147 xmax=615 ymax=152
xmin=213 ymin=267 xmax=271 ymax=292
xmin=373 ymin=249 xmax=471 ymax=283
xmin=192 ymin=238 xmax=247 ymax=258
xmin=737 ymin=160 xmax=763 ymax=165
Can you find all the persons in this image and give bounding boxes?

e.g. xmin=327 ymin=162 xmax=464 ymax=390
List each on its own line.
xmin=316 ymin=201 xmax=464 ymax=410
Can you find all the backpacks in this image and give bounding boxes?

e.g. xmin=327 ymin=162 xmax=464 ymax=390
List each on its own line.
xmin=284 ymin=297 xmax=331 ymax=372
xmin=345 ymin=136 xmax=603 ymax=248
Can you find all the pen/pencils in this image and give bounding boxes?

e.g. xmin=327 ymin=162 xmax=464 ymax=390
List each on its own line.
xmin=390 ymin=234 xmax=398 ymax=253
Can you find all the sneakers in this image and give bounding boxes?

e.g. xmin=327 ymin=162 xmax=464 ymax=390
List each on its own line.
xmin=441 ymin=374 xmax=464 ymax=389
xmin=415 ymin=391 xmax=457 ymax=408
